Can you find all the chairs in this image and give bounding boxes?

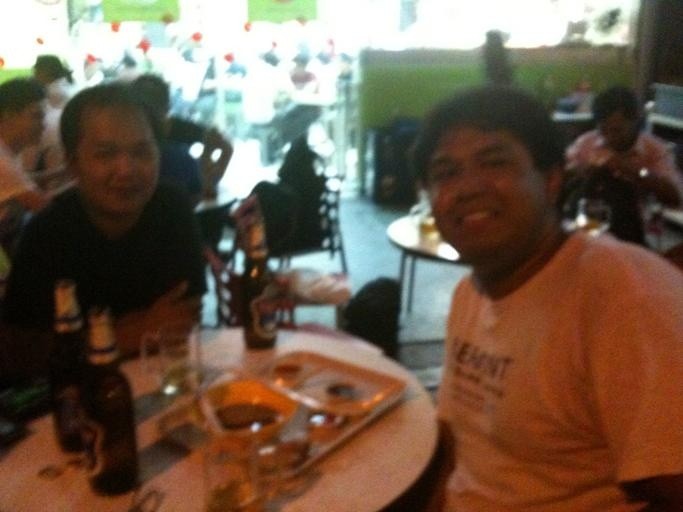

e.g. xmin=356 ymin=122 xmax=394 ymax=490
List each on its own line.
xmin=187 ymin=125 xmax=232 ymax=206
xmin=195 ymin=222 xmax=299 ymax=335
xmin=267 ymin=171 xmax=352 ymax=274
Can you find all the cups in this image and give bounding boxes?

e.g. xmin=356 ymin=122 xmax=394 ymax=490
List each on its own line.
xmin=578 ymin=199 xmax=611 ymax=237
xmin=203 ymin=442 xmax=279 ymax=511
xmin=140 ymin=320 xmax=200 ymax=396
xmin=410 ymin=190 xmax=439 ymax=242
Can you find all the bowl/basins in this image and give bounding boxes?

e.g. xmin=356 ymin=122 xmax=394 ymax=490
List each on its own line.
xmin=200 ymin=379 xmax=297 ymax=454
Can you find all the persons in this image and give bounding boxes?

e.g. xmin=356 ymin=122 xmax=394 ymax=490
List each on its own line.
xmin=290 ymin=53 xmax=314 ymax=86
xmin=565 ymin=87 xmax=682 ymax=208
xmin=32 ymin=53 xmax=73 ymax=84
xmin=134 ymin=74 xmax=234 ymax=195
xmin=0 ymin=83 xmax=209 ymax=377
xmin=240 ymin=53 xmax=330 ymax=130
xmin=134 ymin=123 xmax=219 ymax=206
xmin=414 ymin=84 xmax=682 ymax=511
xmin=224 ymin=103 xmax=324 ymax=275
xmin=1 ymin=76 xmax=78 ymax=229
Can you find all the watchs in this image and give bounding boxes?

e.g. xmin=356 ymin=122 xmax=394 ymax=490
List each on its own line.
xmin=637 ymin=167 xmax=651 ymax=179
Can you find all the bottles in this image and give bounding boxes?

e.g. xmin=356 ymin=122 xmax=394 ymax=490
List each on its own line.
xmin=77 ymin=306 xmax=140 ymax=497
xmin=47 ymin=280 xmax=90 ymax=454
xmin=239 ymin=211 xmax=277 ymax=351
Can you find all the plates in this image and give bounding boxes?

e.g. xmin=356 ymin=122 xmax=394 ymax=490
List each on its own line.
xmin=158 ymin=350 xmax=407 ymax=485
